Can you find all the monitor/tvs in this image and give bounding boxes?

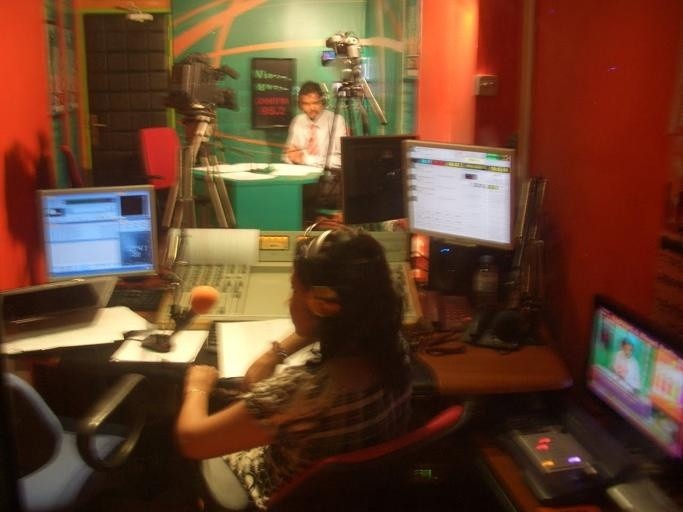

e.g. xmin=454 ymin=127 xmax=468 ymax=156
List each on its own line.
xmin=581 ymin=293 xmax=683 ymax=460
xmin=401 ymin=138 xmax=517 ymax=251
xmin=340 ymin=135 xmax=420 ymax=226
xmin=34 ymin=184 xmax=158 ymax=283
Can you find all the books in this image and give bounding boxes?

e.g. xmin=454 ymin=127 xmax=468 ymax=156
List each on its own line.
xmin=214 ymin=319 xmax=298 ymax=382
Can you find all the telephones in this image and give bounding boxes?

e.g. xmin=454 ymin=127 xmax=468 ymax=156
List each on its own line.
xmin=424 ymin=290 xmax=475 ymax=331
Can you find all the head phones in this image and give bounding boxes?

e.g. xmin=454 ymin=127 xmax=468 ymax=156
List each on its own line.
xmin=298 ymin=82 xmax=329 ymax=110
xmin=293 ymin=217 xmax=352 ymax=318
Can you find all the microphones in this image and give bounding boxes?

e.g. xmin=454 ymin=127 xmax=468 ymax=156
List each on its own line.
xmin=171 ymin=285 xmax=221 ymax=336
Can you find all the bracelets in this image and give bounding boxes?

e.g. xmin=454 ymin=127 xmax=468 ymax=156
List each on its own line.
xmin=182 ymin=388 xmax=214 ymax=397
xmin=271 ymin=341 xmax=287 ymax=363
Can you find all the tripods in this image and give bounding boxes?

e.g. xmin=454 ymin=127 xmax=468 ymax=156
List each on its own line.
xmin=320 ymin=79 xmax=387 ymax=207
xmin=162 ymin=116 xmax=237 ymax=229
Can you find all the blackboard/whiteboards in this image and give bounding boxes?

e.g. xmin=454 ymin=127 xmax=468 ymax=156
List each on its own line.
xmin=250 ymin=58 xmax=297 ymax=129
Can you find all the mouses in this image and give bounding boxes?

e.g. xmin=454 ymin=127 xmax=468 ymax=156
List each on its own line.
xmin=141 ymin=335 xmax=171 ymax=353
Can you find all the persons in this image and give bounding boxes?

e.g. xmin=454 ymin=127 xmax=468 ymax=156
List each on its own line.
xmin=611 ymin=336 xmax=641 ymax=389
xmin=279 ymin=81 xmax=347 ymax=169
xmin=174 ymin=226 xmax=416 ymax=510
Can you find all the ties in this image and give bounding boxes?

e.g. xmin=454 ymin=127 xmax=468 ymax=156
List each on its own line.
xmin=303 ymin=124 xmax=320 ymax=157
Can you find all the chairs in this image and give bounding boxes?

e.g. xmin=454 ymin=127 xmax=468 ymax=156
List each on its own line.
xmin=196 ymin=401 xmax=471 ymax=511
xmin=0 ymin=370 xmax=165 ymax=512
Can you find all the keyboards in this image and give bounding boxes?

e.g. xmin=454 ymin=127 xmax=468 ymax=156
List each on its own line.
xmin=108 ymin=286 xmax=162 ymax=310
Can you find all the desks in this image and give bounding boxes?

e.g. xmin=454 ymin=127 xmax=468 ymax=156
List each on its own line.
xmin=0 ymin=326 xmax=259 ymax=387
xmin=472 ymin=430 xmax=618 ymax=511
xmin=409 ymin=291 xmax=574 ymax=398
xmin=190 ymin=160 xmax=326 ymax=231
